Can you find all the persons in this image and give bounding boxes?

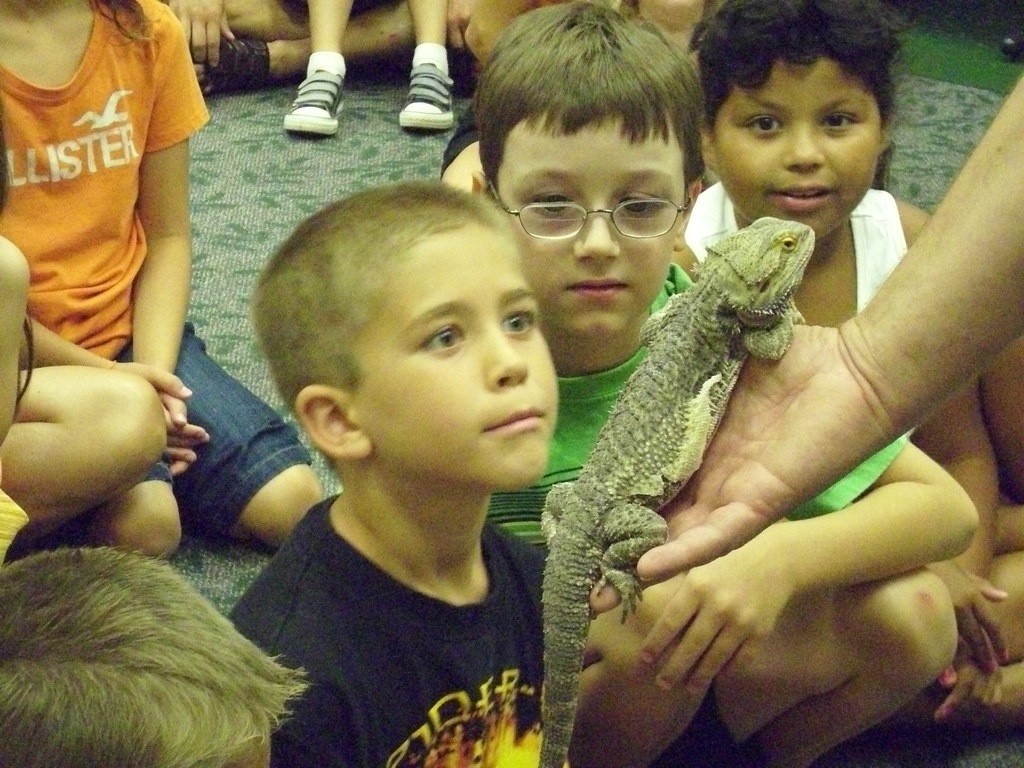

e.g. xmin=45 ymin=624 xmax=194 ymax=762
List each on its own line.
xmin=675 ymin=0 xmax=1024 ymax=740
xmin=2 ymin=0 xmax=322 ymax=566
xmin=282 ymin=0 xmax=452 ymax=137
xmin=169 ymin=0 xmax=480 ymax=98
xmin=477 ymin=6 xmax=982 ymax=765
xmin=216 ymin=179 xmax=561 ymax=768
xmin=0 ymin=542 xmax=307 ymax=767
xmin=588 ymin=71 xmax=1024 ymax=611
xmin=450 ymin=1 xmax=725 ymax=65
xmin=0 ymin=104 xmax=172 ymax=570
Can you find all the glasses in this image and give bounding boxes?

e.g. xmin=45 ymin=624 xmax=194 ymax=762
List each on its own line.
xmin=488 ymin=178 xmax=691 ymax=239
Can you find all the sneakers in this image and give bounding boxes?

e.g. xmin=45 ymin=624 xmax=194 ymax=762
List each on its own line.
xmin=399 ymin=63 xmax=454 ymax=129
xmin=283 ymin=69 xmax=344 ymax=134
